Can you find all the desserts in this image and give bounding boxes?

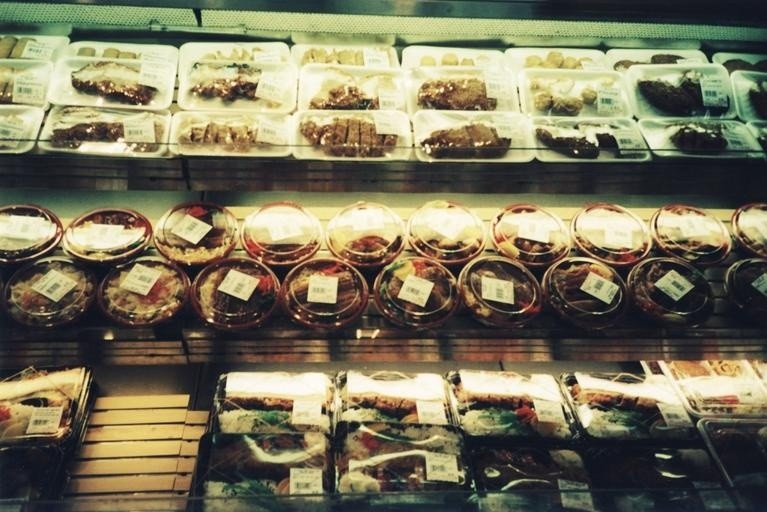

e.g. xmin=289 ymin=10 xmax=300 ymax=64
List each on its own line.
xmin=0 ymin=32 xmax=763 ymax=159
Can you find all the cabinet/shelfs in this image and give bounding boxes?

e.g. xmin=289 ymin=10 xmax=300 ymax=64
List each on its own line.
xmin=0 ymin=150 xmax=766 ymax=366
